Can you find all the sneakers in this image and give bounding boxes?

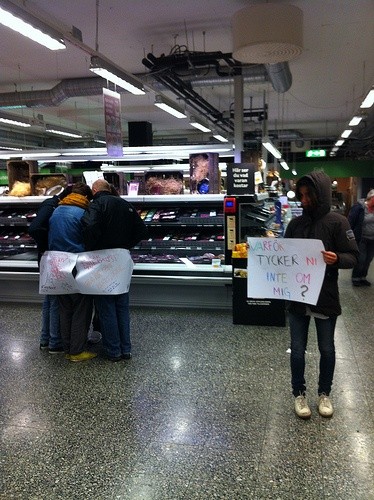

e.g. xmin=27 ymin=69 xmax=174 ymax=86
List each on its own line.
xmin=295 ymin=391 xmax=311 ymax=416
xmin=318 ymin=392 xmax=334 ymax=417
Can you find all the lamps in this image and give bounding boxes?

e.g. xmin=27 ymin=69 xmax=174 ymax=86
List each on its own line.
xmin=263 ymin=141 xmax=297 ymax=177
xmin=0 ymin=0 xmax=235 ymax=164
xmin=330 ymin=85 xmax=374 ymax=159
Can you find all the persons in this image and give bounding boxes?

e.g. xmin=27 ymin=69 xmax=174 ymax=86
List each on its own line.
xmin=246 ymin=172 xmax=360 ymax=417
xmin=30 ymin=180 xmax=146 ymax=362
xmin=352 ymin=190 xmax=374 ymax=287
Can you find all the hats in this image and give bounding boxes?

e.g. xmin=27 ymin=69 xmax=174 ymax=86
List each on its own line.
xmin=367 ymin=190 xmax=374 ymax=199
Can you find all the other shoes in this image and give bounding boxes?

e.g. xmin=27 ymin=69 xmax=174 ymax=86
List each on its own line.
xmin=48 ymin=348 xmax=65 ymax=354
xmin=100 ymin=348 xmax=121 ymax=361
xmin=88 ymin=330 xmax=102 ymax=342
xmin=122 ymin=353 xmax=132 ymax=359
xmin=40 ymin=342 xmax=50 ymax=349
xmin=352 ymin=278 xmax=371 ymax=288
xmin=65 ymin=354 xmax=71 ymax=359
xmin=71 ymin=351 xmax=97 ymax=361
